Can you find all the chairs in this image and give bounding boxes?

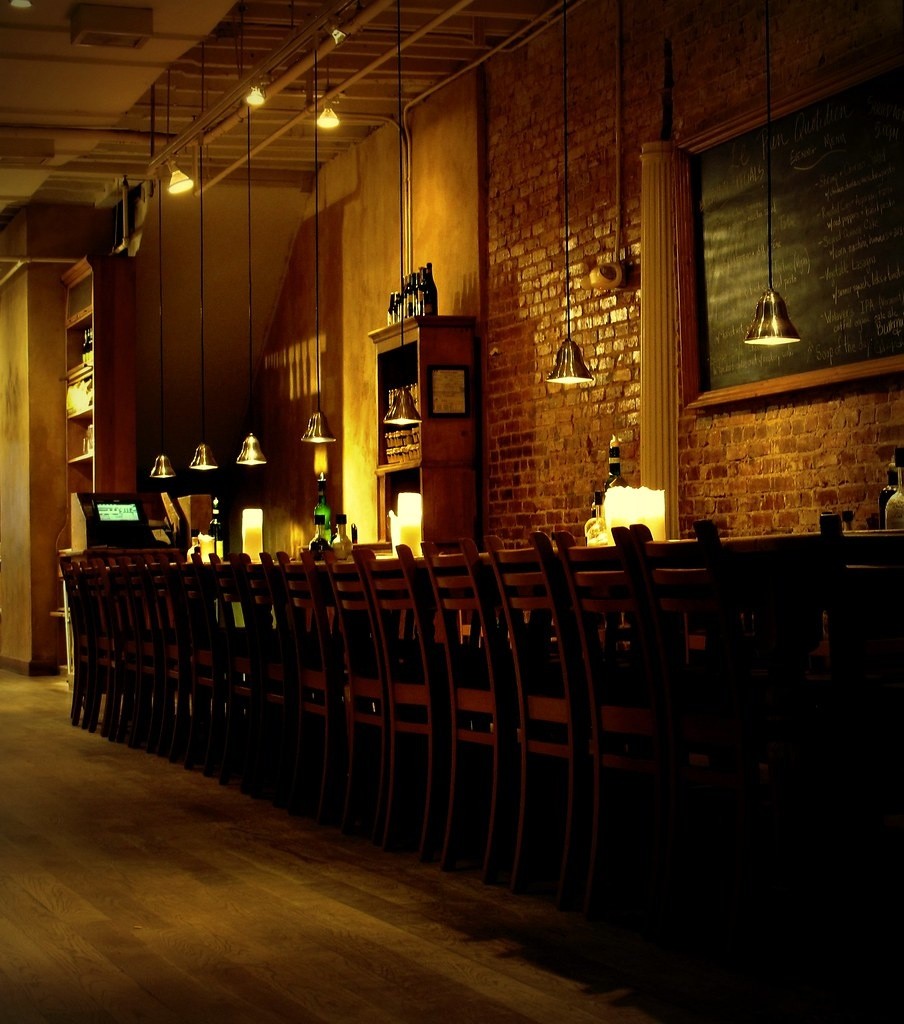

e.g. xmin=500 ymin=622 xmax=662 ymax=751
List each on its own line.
xmin=59 ymin=513 xmax=904 ymax=991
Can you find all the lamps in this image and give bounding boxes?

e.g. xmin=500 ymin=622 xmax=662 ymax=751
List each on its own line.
xmin=189 ymin=130 xmax=219 ymax=470
xmin=744 ymin=1 xmax=801 ymax=347
xmin=245 ymin=83 xmax=266 ymax=107
xmin=383 ymin=0 xmax=422 ymax=425
xmin=328 ymin=25 xmax=350 ymax=45
xmin=150 ymin=181 xmax=176 ymax=478
xmin=545 ymin=1 xmax=594 ymax=384
xmin=236 ymin=106 xmax=266 ymax=465
xmin=163 ymin=156 xmax=195 ymax=196
xmin=301 ymin=46 xmax=336 ymax=443
xmin=317 ymin=64 xmax=339 ymax=130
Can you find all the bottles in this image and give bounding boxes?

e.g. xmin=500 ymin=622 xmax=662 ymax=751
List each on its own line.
xmin=388 ymin=263 xmax=437 ymax=329
xmin=879 ymin=447 xmax=904 ymax=530
xmin=333 ymin=513 xmax=352 ymax=559
xmin=584 ymin=434 xmax=629 ymax=547
xmin=187 ymin=528 xmax=200 ymax=563
xmin=308 ymin=478 xmax=331 ymax=561
xmin=842 ymin=510 xmax=853 ymax=530
xmin=210 ymin=499 xmax=225 ymax=562
xmin=82 ymin=328 xmax=92 ymax=363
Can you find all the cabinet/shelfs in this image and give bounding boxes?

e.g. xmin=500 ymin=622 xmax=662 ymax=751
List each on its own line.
xmin=367 ymin=315 xmax=478 ymax=549
xmin=61 ymin=254 xmax=138 ymax=517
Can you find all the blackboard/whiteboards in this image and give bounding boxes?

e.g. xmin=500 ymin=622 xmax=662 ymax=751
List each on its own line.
xmin=678 ymin=51 xmax=904 ymax=408
